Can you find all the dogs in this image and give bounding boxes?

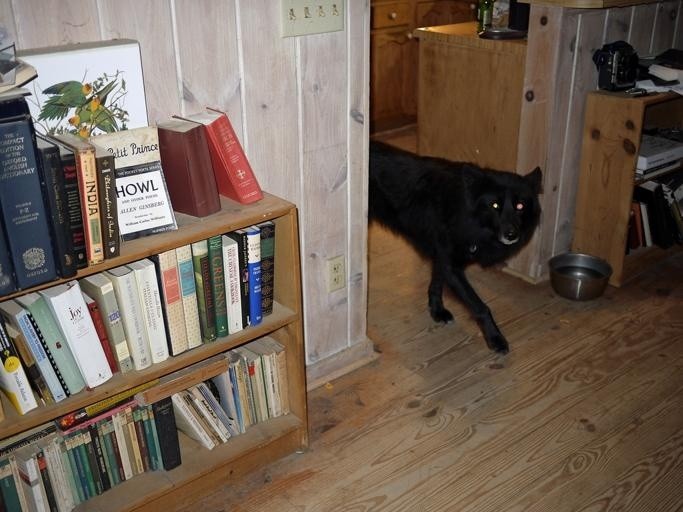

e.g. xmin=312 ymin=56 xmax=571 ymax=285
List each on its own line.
xmin=367 ymin=138 xmax=543 ymax=355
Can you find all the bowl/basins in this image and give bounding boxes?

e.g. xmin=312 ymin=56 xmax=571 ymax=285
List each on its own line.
xmin=548 ymin=252 xmax=613 ymax=299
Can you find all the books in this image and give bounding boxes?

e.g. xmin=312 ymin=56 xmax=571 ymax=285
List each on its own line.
xmin=624 ymin=128 xmax=683 ymax=257
xmin=0 ymin=219 xmax=274 ymax=422
xmin=1 ymin=97 xmax=263 ymax=301
xmin=0 ymin=336 xmax=289 ymax=512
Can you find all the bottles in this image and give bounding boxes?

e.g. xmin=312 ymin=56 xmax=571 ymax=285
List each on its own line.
xmin=477 ymin=0 xmax=494 ymax=34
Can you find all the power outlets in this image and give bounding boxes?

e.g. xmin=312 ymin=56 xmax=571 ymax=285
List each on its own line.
xmin=327 ymin=254 xmax=346 ymax=293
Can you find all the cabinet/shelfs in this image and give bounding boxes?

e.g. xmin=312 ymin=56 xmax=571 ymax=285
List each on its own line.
xmin=0 ymin=188 xmax=309 ymax=512
xmin=414 ymin=38 xmax=527 ymax=172
xmin=569 ymin=78 xmax=683 ymax=287
xmin=370 ymin=0 xmax=479 ymax=133
